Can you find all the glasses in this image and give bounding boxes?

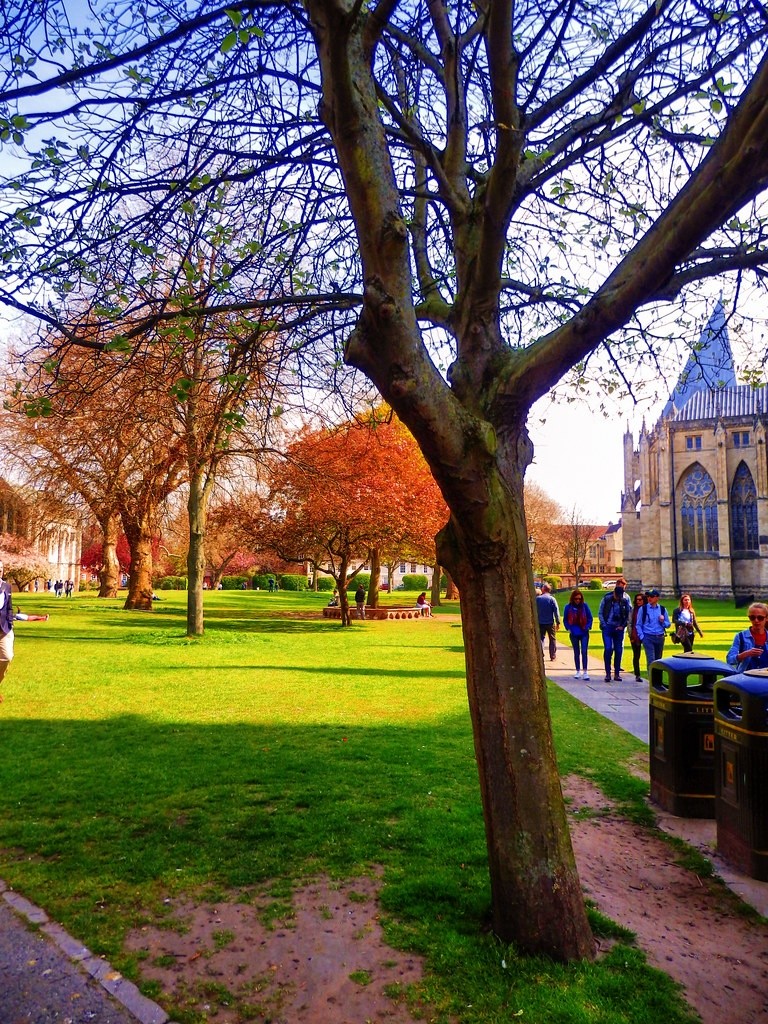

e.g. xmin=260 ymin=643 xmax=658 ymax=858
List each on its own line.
xmin=636 ymin=599 xmax=643 ymax=601
xmin=748 ymin=614 xmax=767 ymax=621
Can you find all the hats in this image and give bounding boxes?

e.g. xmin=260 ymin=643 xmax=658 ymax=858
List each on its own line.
xmin=645 ymin=590 xmax=660 ymax=596
xmin=615 ymin=586 xmax=624 ymax=598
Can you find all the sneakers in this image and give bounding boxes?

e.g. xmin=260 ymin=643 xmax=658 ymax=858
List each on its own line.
xmin=574 ymin=671 xmax=582 ymax=679
xmin=582 ymin=672 xmax=590 ymax=680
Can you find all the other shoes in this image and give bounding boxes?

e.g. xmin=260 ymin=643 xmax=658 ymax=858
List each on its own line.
xmin=636 ymin=676 xmax=643 ymax=682
xmin=614 ymin=675 xmax=622 ymax=681
xmin=614 ymin=667 xmax=624 ymax=671
xmin=604 ymin=673 xmax=611 ymax=682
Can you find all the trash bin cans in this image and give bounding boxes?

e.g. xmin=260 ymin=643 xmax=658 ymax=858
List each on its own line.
xmin=711 ymin=667 xmax=768 ymax=883
xmin=646 ymin=652 xmax=746 ymax=819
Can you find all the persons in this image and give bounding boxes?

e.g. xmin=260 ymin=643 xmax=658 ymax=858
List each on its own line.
xmin=12 ymin=606 xmax=50 ymax=622
xmin=598 ymin=586 xmax=630 ymax=681
xmin=46 ymin=578 xmax=76 ymax=600
xmin=535 ymin=589 xmax=542 ymax=596
xmin=35 ymin=578 xmax=39 ymax=592
xmin=726 ymin=602 xmax=768 ymax=674
xmin=672 ymin=595 xmax=703 ymax=653
xmin=330 ymin=589 xmax=340 ymax=606
xmin=627 ymin=593 xmax=647 ymax=682
xmin=536 ymin=583 xmax=561 ymax=662
xmin=0 ymin=561 xmax=15 ymax=682
xmin=268 ymin=578 xmax=274 ymax=592
xmin=636 ymin=590 xmax=671 ymax=673
xmin=355 ymin=584 xmax=367 ymax=620
xmin=614 ymin=578 xmax=633 ymax=672
xmin=275 ymin=582 xmax=279 ymax=591
xmin=151 ymin=592 xmax=161 ymax=600
xmin=563 ymin=590 xmax=593 ymax=680
xmin=416 ymin=592 xmax=433 ymax=617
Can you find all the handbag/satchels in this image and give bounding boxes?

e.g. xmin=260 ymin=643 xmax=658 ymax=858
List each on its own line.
xmin=670 ymin=625 xmax=688 ymax=644
